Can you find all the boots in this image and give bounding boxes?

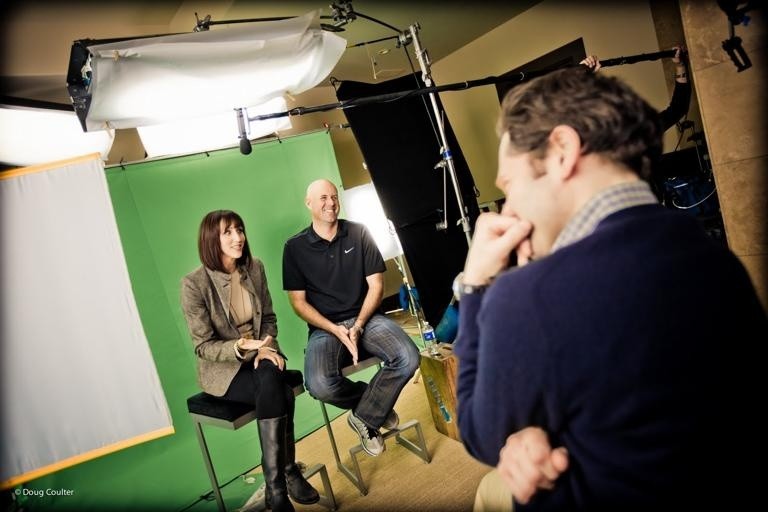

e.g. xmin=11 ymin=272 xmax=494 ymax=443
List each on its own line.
xmin=257 ymin=415 xmax=320 ymax=512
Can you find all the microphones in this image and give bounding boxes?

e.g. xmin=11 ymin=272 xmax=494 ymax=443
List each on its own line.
xmin=235 ymin=109 xmax=253 ymax=158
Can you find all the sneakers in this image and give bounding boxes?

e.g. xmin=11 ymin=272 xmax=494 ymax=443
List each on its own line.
xmin=357 ymin=381 xmax=399 ymax=430
xmin=348 ymin=409 xmax=385 ymax=456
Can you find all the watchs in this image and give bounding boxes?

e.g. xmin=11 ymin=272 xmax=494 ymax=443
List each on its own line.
xmin=452 ymin=271 xmax=489 ymax=302
xmin=354 ymin=325 xmax=365 ymax=336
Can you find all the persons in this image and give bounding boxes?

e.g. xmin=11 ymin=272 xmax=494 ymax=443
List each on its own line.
xmin=453 ymin=66 xmax=768 ymax=512
xmin=179 ymin=208 xmax=321 ymax=511
xmin=281 ymin=178 xmax=421 ymax=457
xmin=577 ymin=45 xmax=693 ymax=163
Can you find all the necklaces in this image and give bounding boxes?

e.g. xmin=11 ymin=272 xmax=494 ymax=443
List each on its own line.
xmin=229 ymin=267 xmax=254 ymax=340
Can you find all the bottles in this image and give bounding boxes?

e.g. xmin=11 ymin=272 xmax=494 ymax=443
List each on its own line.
xmin=421 ymin=321 xmax=439 ymax=356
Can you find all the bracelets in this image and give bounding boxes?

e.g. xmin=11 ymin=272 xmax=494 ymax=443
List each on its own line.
xmin=676 ymin=72 xmax=685 ymax=80
xmin=236 ymin=342 xmax=250 ymax=353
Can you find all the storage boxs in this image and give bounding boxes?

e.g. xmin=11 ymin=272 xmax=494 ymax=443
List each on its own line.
xmin=418 ymin=339 xmax=466 ymax=441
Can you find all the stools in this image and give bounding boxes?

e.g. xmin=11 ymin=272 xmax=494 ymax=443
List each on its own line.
xmin=184 ymin=343 xmax=432 ymax=511
